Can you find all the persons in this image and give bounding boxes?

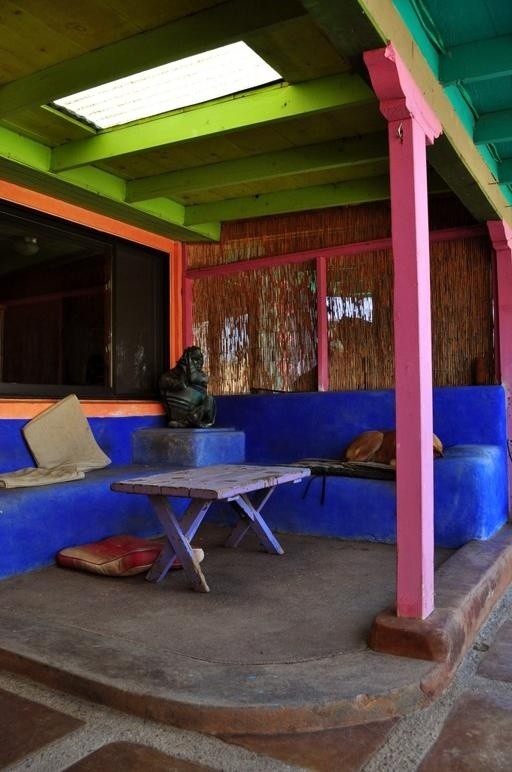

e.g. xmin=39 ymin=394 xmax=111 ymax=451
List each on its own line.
xmin=159 ymin=346 xmax=217 ymax=428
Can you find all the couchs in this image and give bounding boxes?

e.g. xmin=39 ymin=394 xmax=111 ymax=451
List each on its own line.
xmin=0 ymin=385 xmax=511 ymax=582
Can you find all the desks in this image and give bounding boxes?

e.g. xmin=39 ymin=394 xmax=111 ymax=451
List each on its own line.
xmin=108 ymin=463 xmax=312 ymax=592
